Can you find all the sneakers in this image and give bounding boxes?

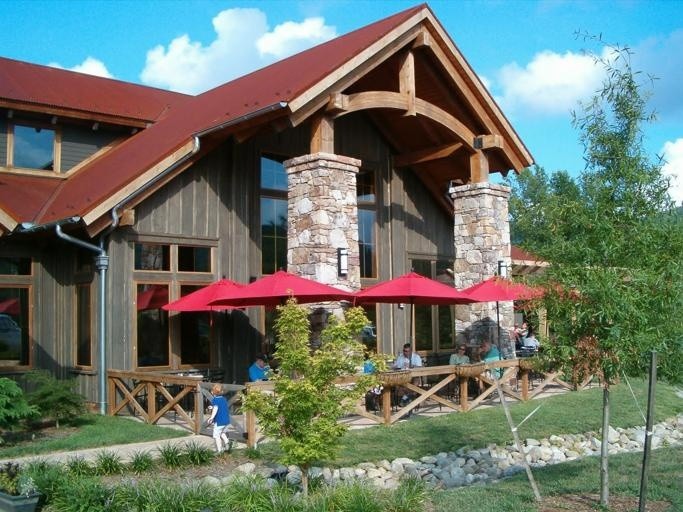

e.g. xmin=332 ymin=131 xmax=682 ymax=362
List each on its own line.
xmin=224 ymin=442 xmax=231 ymax=452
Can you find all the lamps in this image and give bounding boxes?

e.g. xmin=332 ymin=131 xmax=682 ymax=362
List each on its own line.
xmin=337 ymin=246 xmax=348 ymax=276
xmin=497 ymin=259 xmax=507 ymax=278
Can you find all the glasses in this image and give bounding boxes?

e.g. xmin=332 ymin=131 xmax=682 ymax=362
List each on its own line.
xmin=459 ymin=347 xmax=466 ymax=351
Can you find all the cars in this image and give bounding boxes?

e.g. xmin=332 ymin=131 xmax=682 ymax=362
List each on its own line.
xmin=514 ymin=329 xmax=538 ymax=356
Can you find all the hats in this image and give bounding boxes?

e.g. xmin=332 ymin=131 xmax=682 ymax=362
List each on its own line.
xmin=255 ymin=353 xmax=267 ymax=360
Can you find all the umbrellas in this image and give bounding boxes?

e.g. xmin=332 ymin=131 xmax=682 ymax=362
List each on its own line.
xmin=137 ymin=285 xmax=168 ymax=361
xmin=353 ymin=270 xmax=477 ymax=371
xmin=163 ymin=273 xmax=244 ymax=384
xmin=1 ymin=300 xmax=20 ymax=318
xmin=462 ymin=272 xmax=543 ymax=360
xmin=209 ymin=267 xmax=359 ymax=381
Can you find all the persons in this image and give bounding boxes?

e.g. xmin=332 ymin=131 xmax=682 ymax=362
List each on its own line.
xmin=364 ymin=349 xmax=377 ymax=374
xmin=516 ymin=320 xmax=532 ymax=349
xmin=393 ymin=344 xmax=423 ymax=407
xmin=207 ymin=384 xmax=234 ymax=455
xmin=248 ymin=352 xmax=274 ymax=382
xmin=476 ymin=340 xmax=504 ymax=394
xmin=448 ymin=343 xmax=471 ymax=397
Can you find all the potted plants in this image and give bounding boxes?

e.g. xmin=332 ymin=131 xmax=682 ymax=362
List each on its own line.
xmin=0 ymin=466 xmax=42 ymax=511
